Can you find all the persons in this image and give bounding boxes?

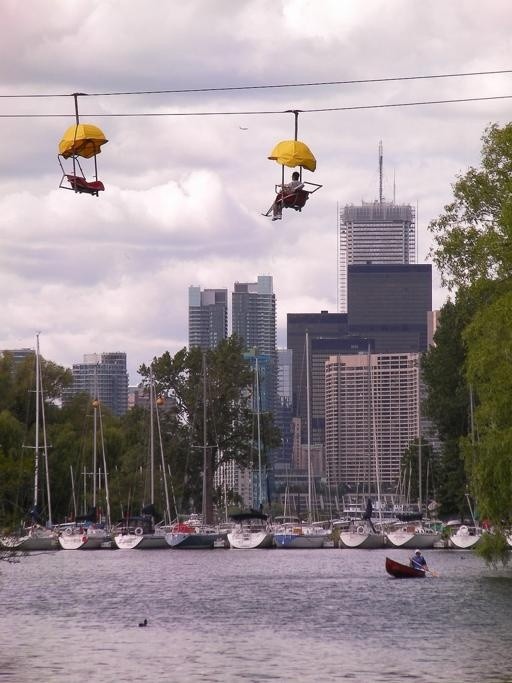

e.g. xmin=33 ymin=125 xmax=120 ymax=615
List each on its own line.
xmin=271 ymin=171 xmax=301 ymax=220
xmin=409 ymin=548 xmax=429 ymax=572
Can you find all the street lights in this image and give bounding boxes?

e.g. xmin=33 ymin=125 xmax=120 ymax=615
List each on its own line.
xmin=93 ymin=399 xmax=99 ymax=508
xmin=156 ymin=392 xmax=171 ymax=526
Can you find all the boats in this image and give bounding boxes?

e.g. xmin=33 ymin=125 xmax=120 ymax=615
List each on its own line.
xmin=386 ymin=557 xmax=426 ymax=577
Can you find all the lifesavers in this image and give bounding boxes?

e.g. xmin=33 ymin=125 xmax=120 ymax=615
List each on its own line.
xmin=357 ymin=526 xmax=366 ymax=535
xmin=134 ymin=527 xmax=143 ymax=536
xmin=65 ymin=528 xmax=73 ymax=536
xmin=460 ymin=525 xmax=468 ymax=534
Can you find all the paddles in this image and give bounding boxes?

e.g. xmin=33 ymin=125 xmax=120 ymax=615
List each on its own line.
xmin=408 ymin=556 xmax=439 ymax=577
